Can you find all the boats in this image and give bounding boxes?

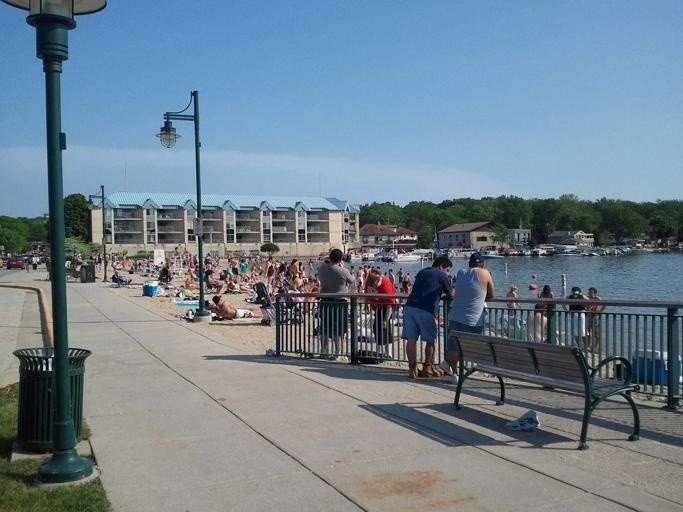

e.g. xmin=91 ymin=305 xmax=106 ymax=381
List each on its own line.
xmin=481 ymin=255 xmax=505 ymax=259
xmin=580 ymin=247 xmax=624 ymax=257
xmin=364 ymin=249 xmax=421 ymax=261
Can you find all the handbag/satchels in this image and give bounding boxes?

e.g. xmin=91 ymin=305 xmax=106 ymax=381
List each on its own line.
xmin=357 ymin=350 xmax=391 ymax=364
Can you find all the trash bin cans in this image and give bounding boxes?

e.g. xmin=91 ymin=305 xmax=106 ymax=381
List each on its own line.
xmin=81 ymin=260 xmax=95 ymax=283
xmin=13 ymin=347 xmax=92 ymax=454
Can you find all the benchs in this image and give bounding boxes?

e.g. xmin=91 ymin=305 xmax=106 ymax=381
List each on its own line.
xmin=448 ymin=329 xmax=642 ymax=450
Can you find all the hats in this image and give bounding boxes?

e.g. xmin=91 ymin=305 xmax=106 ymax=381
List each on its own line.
xmin=470 ymin=253 xmax=484 ymax=263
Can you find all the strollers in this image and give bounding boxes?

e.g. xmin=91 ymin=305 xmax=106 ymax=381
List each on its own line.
xmin=158 ymin=268 xmax=172 ymax=282
xmin=253 ymin=283 xmax=304 ymax=327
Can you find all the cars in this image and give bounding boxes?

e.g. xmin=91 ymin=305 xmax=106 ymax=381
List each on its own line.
xmin=7 ymin=256 xmax=25 ymax=269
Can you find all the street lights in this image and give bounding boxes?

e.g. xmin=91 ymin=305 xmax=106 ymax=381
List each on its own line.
xmin=1 ymin=0 xmax=109 ymax=483
xmin=155 ymin=90 xmax=210 ymax=317
xmin=43 ymin=213 xmax=50 ymax=224
xmin=86 ymin=185 xmax=108 ymax=282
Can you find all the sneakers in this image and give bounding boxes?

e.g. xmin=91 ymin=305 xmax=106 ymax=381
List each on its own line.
xmin=410 ymin=360 xmax=459 ymax=384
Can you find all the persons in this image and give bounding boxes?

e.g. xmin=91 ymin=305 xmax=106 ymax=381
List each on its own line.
xmin=24 ymin=256 xmax=30 ymax=272
xmin=173 ymin=246 xmax=320 ymax=320
xmin=318 ymin=244 xmax=606 ymax=385
xmin=32 ymin=254 xmax=38 ymax=270
xmin=65 ymin=247 xmax=175 ymax=288
